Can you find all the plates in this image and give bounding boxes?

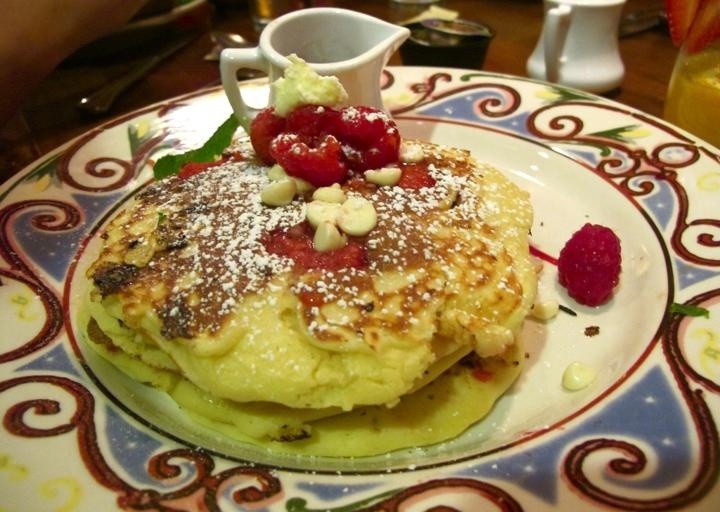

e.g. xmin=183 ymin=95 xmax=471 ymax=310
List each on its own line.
xmin=1 ymin=66 xmax=720 ymax=512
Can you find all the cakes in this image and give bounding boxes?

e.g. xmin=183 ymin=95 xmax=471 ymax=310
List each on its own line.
xmin=77 ymin=53 xmax=540 ymax=457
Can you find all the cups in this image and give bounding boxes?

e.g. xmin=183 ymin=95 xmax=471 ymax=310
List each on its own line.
xmin=521 ymin=0 xmax=629 ymax=92
xmin=224 ymin=8 xmax=496 ymax=113
